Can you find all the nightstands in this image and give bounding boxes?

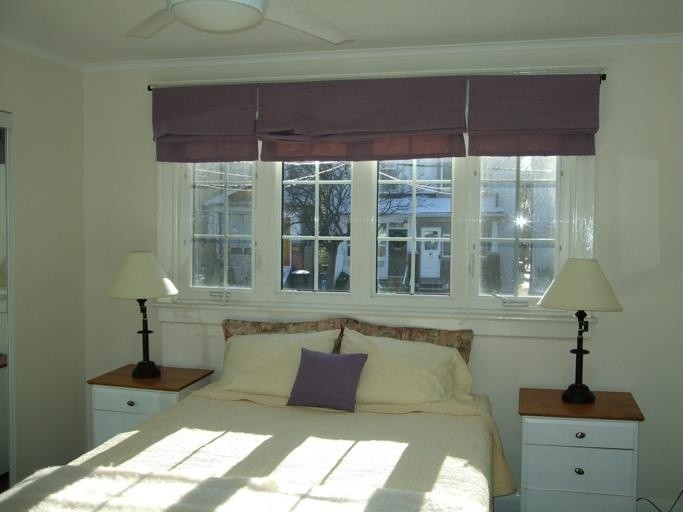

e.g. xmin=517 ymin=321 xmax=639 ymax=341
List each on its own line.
xmin=518 ymin=388 xmax=645 ymax=512
xmin=86 ymin=364 xmax=216 ymax=449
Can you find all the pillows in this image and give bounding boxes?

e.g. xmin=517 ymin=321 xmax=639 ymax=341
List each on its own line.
xmin=285 ymin=348 xmax=369 ymax=413
xmin=191 ymin=329 xmax=341 ymax=407
xmin=340 ymin=326 xmax=475 ymax=414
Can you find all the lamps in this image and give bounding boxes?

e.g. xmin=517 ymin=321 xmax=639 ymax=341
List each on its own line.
xmin=535 ymin=258 xmax=624 ymax=404
xmin=104 ymin=251 xmax=180 ymax=378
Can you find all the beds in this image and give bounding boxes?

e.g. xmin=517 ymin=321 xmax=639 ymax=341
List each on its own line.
xmin=0 ymin=318 xmax=491 ymax=512
